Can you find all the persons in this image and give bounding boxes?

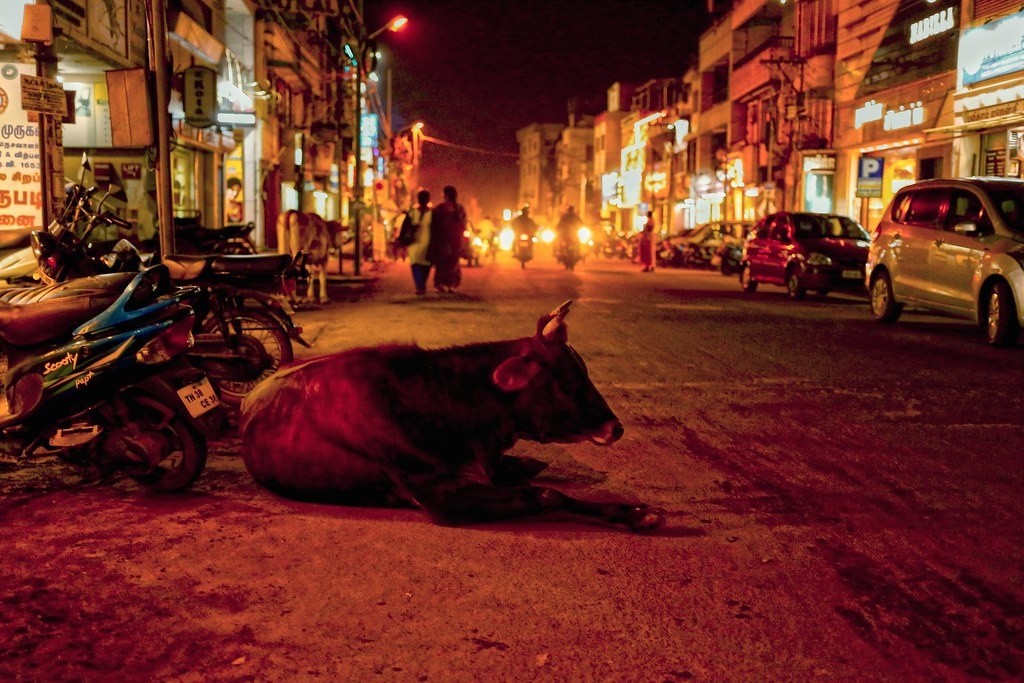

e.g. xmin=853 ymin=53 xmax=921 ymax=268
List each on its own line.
xmin=638 ymin=211 xmax=656 ymax=272
xmin=510 ymin=207 xmax=539 ymax=250
xmin=403 ymin=190 xmax=431 ymax=295
xmin=478 ymin=216 xmax=497 ymax=241
xmin=558 ymin=206 xmax=581 ymax=231
xmin=430 ymin=185 xmax=466 ymax=294
xmin=225 ymin=177 xmax=242 ymax=223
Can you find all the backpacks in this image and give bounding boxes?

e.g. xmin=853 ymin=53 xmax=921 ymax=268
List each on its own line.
xmin=397 ymin=222 xmax=418 ymax=247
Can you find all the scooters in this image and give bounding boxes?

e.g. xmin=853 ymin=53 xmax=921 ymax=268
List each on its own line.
xmin=665 ymin=240 xmax=715 ymax=267
xmin=603 ymin=233 xmax=641 ymax=260
xmin=713 ymin=232 xmax=745 ymax=275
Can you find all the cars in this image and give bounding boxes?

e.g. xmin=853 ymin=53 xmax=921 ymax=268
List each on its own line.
xmin=670 ymin=221 xmax=755 ymax=259
xmin=865 ymin=178 xmax=1024 ymax=345
xmin=740 ymin=210 xmax=873 ymax=299
xmin=459 ymin=221 xmax=483 ymax=266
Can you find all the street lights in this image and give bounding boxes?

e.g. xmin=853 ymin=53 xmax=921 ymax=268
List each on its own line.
xmin=354 ymin=14 xmax=410 ymax=275
xmin=394 ymin=120 xmax=425 ymax=205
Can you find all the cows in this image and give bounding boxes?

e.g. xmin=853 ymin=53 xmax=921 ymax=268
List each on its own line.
xmin=239 ymin=299 xmax=667 ymax=534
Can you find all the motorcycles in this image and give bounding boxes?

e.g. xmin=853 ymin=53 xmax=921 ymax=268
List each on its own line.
xmin=0 ymin=261 xmax=231 ymax=491
xmin=174 ymin=216 xmax=257 ymax=257
xmin=489 ymin=234 xmax=499 ymax=260
xmin=31 ymin=153 xmax=312 ymax=409
xmin=556 ymin=228 xmax=585 ymax=269
xmin=514 ymin=232 xmax=533 ymax=267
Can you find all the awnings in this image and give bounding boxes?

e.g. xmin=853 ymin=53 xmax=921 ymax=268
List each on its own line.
xmin=922 ymin=112 xmax=1024 ymax=133
